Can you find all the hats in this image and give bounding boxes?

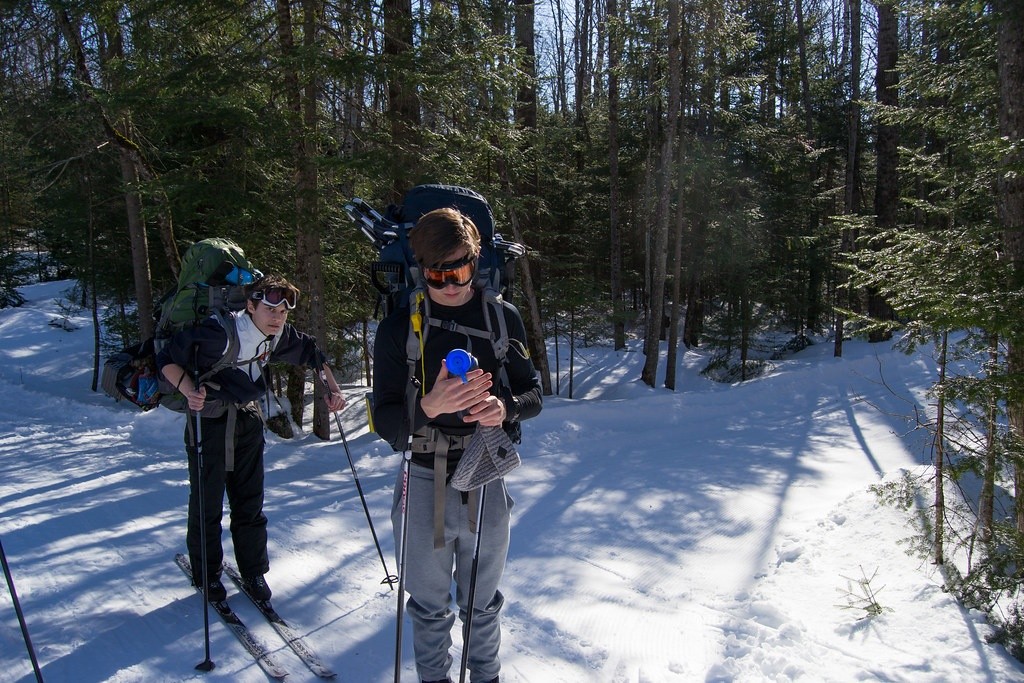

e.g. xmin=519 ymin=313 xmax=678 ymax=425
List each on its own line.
xmin=451 ymin=420 xmax=521 ymax=492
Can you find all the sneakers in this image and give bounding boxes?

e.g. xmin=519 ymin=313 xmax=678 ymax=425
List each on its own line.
xmin=195 ymin=574 xmax=227 ymax=602
xmin=245 ymin=576 xmax=273 ymax=601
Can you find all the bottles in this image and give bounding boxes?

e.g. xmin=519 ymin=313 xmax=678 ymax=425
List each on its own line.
xmin=446 ymin=349 xmax=479 ymax=418
xmin=138 ymin=373 xmax=154 ymax=404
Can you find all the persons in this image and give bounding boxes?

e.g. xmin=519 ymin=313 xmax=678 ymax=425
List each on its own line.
xmin=153 ymin=273 xmax=347 ymax=603
xmin=373 ymin=208 xmax=544 ymax=683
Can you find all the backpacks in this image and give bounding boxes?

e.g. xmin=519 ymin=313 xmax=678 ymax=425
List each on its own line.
xmin=155 ymin=238 xmax=264 ymax=413
xmin=374 ymin=185 xmax=509 ymax=369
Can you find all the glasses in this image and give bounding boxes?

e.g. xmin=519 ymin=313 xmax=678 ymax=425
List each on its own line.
xmin=420 ymin=256 xmax=478 ymax=291
xmin=261 ymin=288 xmax=298 ymax=310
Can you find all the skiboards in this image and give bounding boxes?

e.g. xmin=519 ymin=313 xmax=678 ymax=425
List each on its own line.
xmin=173 ymin=553 xmax=338 ymax=679
